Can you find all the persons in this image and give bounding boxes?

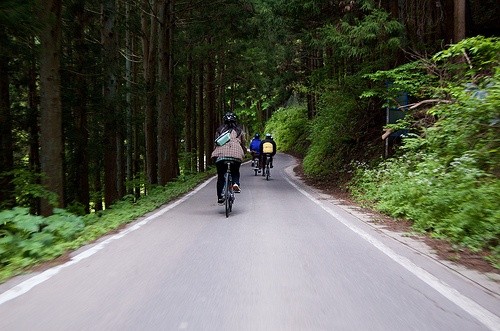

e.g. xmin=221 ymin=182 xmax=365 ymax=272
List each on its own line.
xmin=210 ymin=111 xmax=247 ymax=206
xmin=249 ymin=133 xmax=277 ymax=177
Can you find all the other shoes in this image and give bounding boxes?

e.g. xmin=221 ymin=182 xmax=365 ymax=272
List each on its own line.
xmin=218 ymin=198 xmax=225 ymax=203
xmin=258 ymin=170 xmax=261 ymax=173
xmin=262 ymin=174 xmax=265 ymax=176
xmin=252 ymin=163 xmax=254 ymax=167
xmin=270 ymin=166 xmax=273 ymax=168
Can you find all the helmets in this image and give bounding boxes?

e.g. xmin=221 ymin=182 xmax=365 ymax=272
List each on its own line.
xmin=223 ymin=111 xmax=238 ymax=122
xmin=266 ymin=134 xmax=271 ymax=137
xmin=255 ymin=134 xmax=259 ymax=137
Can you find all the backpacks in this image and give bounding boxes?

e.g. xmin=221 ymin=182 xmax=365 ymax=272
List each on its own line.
xmin=263 ymin=141 xmax=273 ymax=154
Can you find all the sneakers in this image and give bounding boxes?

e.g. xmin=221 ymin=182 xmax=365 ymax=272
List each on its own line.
xmin=233 ymin=182 xmax=241 ymax=191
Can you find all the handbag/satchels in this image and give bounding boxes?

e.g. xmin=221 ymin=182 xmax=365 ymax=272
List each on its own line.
xmin=215 ymin=131 xmax=230 ymax=147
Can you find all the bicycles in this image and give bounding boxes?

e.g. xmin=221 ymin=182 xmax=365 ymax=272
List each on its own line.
xmin=252 ymin=154 xmax=262 ymax=176
xmin=218 ymin=161 xmax=240 ymax=218
xmin=264 ymin=154 xmax=273 ymax=180
